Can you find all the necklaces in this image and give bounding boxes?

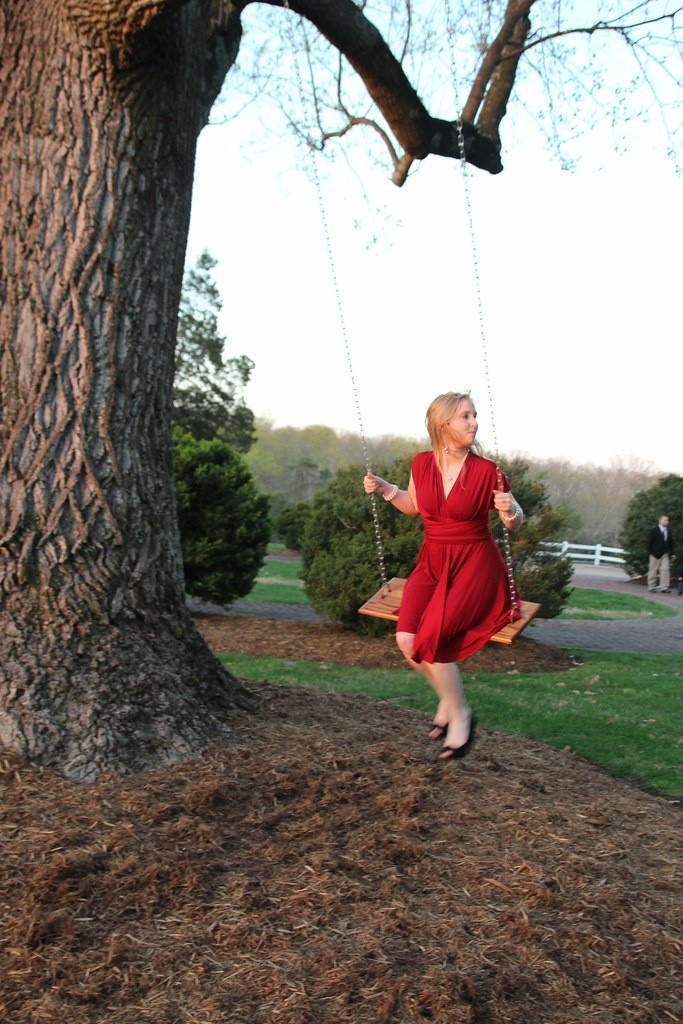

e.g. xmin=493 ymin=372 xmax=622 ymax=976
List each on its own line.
xmin=445 ymin=470 xmax=456 ymax=483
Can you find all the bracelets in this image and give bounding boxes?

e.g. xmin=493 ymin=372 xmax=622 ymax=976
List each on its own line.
xmin=383 ymin=484 xmax=400 ymax=502
xmin=501 ymin=501 xmax=520 ymax=522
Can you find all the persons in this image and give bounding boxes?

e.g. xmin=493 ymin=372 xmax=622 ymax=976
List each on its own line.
xmin=362 ymin=391 xmax=526 ymax=762
xmin=647 ymin=516 xmax=676 ymax=594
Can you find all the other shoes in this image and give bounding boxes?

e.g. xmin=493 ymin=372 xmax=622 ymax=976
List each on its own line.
xmin=428 ymin=719 xmax=448 ymax=739
xmin=661 ymin=589 xmax=671 ymax=593
xmin=648 ymin=589 xmax=657 ymax=593
xmin=442 ymin=708 xmax=477 ymax=758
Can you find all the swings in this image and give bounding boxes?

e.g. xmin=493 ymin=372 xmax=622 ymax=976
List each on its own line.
xmin=287 ymin=0 xmax=544 ymax=648
